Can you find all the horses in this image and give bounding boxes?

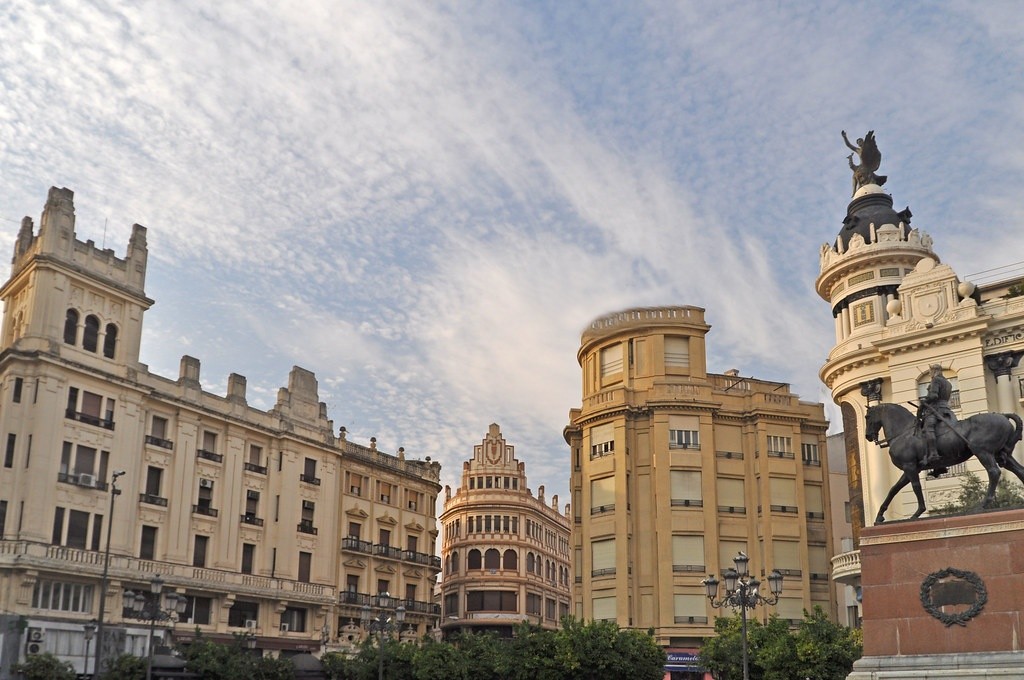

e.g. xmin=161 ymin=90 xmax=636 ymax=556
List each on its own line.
xmin=862 ymin=404 xmax=1024 ymax=526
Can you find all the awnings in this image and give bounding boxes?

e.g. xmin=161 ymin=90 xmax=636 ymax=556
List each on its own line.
xmin=665 ymin=664 xmax=708 ymax=672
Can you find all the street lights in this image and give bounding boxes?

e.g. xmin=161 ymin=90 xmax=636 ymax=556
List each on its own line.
xmin=93 ymin=470 xmax=126 ymax=680
xmin=83 ymin=623 xmax=96 ymax=680
xmin=703 ymin=552 xmax=783 ymax=680
xmin=360 ymin=591 xmax=407 ymax=680
xmin=122 ymin=574 xmax=188 ymax=680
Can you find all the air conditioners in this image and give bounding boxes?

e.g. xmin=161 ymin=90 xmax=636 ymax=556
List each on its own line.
xmin=77 ymin=473 xmax=95 ymax=487
xmin=26 ymin=643 xmax=44 ymax=654
xmin=199 ymin=478 xmax=212 ymax=488
xmin=281 ymin=623 xmax=288 ymax=632
xmin=303 ymin=500 xmax=313 ymax=509
xmin=245 ymin=620 xmax=257 ymax=628
xmin=30 ymin=630 xmax=44 ymax=641
xmin=247 ymin=491 xmax=258 ymax=499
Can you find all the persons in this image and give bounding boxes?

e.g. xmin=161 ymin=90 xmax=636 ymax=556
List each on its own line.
xmin=917 ymin=364 xmax=959 ymax=477
xmin=841 ymin=129 xmax=865 ymax=198
